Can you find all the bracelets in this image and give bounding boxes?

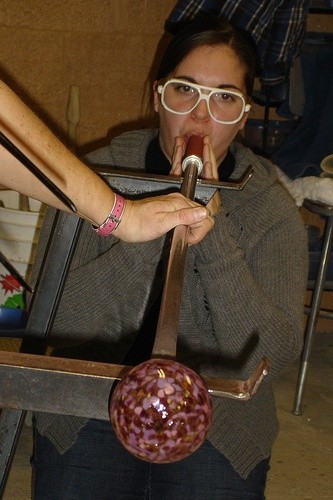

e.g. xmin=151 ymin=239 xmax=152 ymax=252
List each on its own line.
xmin=92 ymin=193 xmax=125 ymax=237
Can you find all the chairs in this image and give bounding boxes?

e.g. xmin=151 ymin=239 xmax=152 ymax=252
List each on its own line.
xmin=269 ymin=0 xmax=333 ymax=414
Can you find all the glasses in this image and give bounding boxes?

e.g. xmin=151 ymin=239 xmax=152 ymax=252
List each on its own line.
xmin=157 ymin=77 xmax=251 ymax=125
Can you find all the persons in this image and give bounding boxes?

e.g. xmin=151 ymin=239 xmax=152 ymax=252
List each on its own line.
xmin=28 ymin=14 xmax=308 ymax=500
xmin=1 ymin=82 xmax=214 ymax=245
